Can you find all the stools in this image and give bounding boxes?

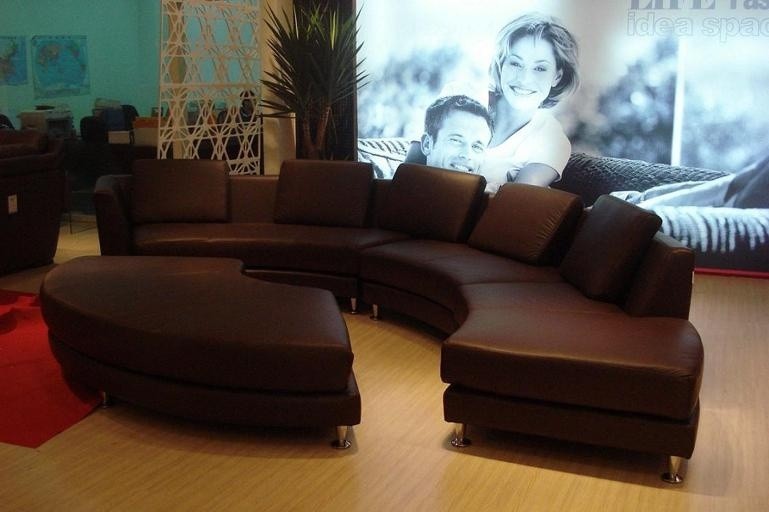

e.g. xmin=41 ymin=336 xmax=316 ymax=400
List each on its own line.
xmin=438 ymin=311 xmax=704 ymax=485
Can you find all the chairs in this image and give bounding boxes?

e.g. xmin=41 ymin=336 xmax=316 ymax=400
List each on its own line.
xmin=0 ymin=91 xmax=256 ymax=233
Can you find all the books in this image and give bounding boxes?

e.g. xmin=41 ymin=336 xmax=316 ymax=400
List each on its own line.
xmin=107 ymin=116 xmax=164 ymax=147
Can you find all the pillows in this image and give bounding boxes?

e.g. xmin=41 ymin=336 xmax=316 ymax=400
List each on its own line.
xmin=274 ymin=158 xmax=373 ymax=227
xmin=560 ymin=195 xmax=662 ymax=301
xmin=377 ymin=163 xmax=487 ymax=243
xmin=468 ymin=182 xmax=585 ymax=266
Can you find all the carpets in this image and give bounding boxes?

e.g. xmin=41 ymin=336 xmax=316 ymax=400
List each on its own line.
xmin=0 ymin=288 xmax=105 ymax=449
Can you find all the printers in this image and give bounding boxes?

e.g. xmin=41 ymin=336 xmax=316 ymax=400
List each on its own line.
xmin=20 ymin=105 xmax=73 ymax=131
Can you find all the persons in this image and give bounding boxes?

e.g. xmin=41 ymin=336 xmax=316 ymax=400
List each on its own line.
xmin=234 ymin=91 xmax=258 ymax=125
xmin=403 ymin=14 xmax=583 ymax=199
xmin=420 ymin=94 xmax=494 ymax=175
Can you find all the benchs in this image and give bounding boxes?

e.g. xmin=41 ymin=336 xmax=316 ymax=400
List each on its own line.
xmin=38 ymin=254 xmax=362 ymax=450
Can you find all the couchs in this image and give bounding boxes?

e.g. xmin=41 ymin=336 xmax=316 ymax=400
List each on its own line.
xmin=95 ymin=176 xmax=695 ymax=321
